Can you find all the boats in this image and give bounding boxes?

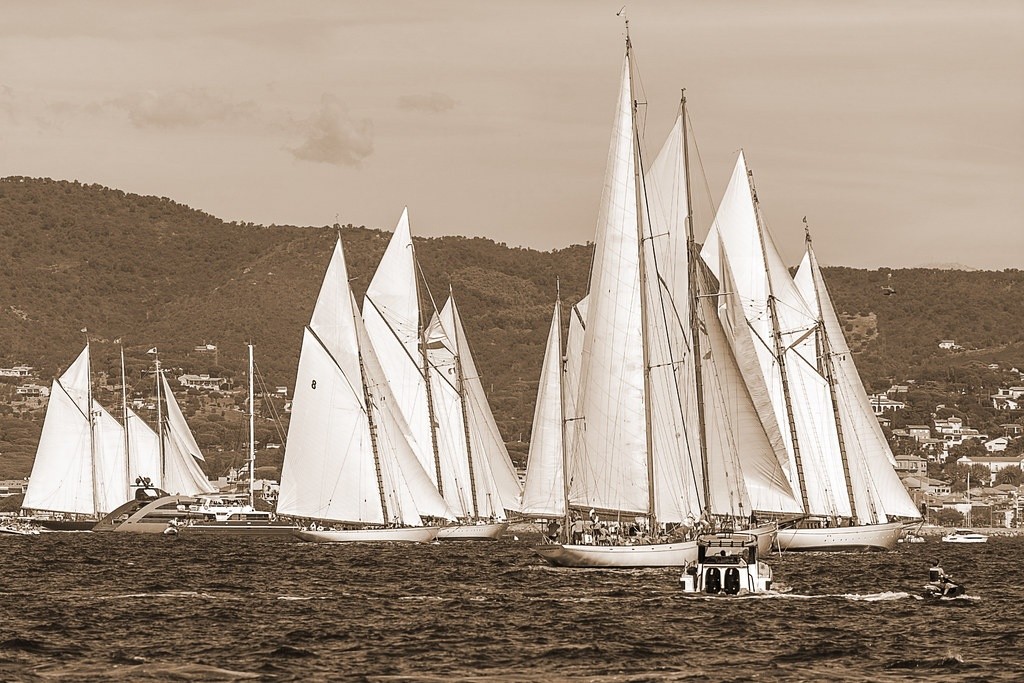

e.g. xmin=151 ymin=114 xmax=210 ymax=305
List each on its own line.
xmin=942 ymin=529 xmax=990 ymax=543
xmin=919 ymin=563 xmax=982 ymax=607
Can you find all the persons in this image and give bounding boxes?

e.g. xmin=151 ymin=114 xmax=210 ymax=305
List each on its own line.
xmin=185 ymin=510 xmax=209 ymax=526
xmin=466 ymin=512 xmax=472 ymax=526
xmin=573 ymin=516 xmax=583 ymax=545
xmin=836 ymin=515 xmax=842 ymax=528
xmin=392 ymin=516 xmax=399 ymax=529
xmin=427 ymin=514 xmax=435 ymax=527
xmin=300 ymin=520 xmax=402 ymax=531
xmin=747 ymin=512 xmax=758 ymax=529
xmin=699 ymin=506 xmax=710 ymax=533
xmin=930 ymin=559 xmax=953 ymax=586
xmin=718 ymin=550 xmax=729 ymax=563
xmin=571 ymin=521 xmax=665 ymax=546
xmin=548 ymin=519 xmax=560 ymax=543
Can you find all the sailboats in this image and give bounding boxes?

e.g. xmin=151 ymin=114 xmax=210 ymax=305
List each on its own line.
xmin=16 ymin=326 xmax=302 ymax=535
xmin=519 ymin=1 xmax=926 ymax=596
xmin=275 ymin=204 xmax=526 ymax=542
